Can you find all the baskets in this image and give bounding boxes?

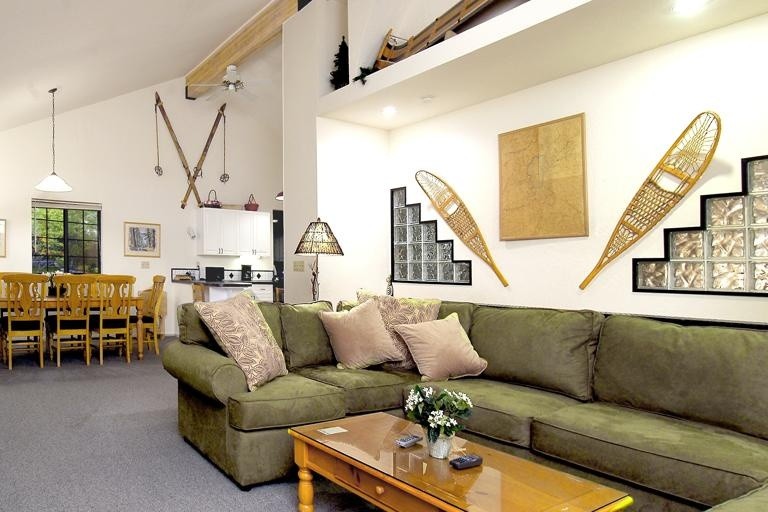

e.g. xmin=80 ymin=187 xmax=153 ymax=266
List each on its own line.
xmin=245 ymin=194 xmax=258 ymax=211
xmin=204 ymin=190 xmax=221 ymax=208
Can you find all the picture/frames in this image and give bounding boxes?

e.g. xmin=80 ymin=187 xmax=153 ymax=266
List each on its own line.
xmin=123 ymin=221 xmax=161 ymax=259
xmin=0 ymin=218 xmax=7 ymax=259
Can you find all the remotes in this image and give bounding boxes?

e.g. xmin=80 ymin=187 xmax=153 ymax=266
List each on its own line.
xmin=395 ymin=434 xmax=423 ymax=448
xmin=450 ymin=454 xmax=482 ymax=469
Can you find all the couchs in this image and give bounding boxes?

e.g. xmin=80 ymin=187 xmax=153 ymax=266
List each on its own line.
xmin=159 ymin=299 xmax=768 ymax=512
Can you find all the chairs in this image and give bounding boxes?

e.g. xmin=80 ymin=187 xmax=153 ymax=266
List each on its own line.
xmin=0 ymin=270 xmax=168 ymax=372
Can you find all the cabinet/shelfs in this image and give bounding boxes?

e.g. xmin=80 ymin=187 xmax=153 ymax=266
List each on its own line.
xmin=195 ymin=208 xmax=272 ymax=258
xmin=206 ymin=286 xmax=274 ymax=305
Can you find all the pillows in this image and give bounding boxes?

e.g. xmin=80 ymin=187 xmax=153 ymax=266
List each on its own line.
xmin=192 ymin=289 xmax=292 ymax=392
xmin=314 ymin=289 xmax=490 ymax=389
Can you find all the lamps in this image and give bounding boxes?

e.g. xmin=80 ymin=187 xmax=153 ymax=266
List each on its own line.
xmin=294 ymin=220 xmax=340 ymax=302
xmin=184 ymin=64 xmax=247 ymax=93
xmin=274 ymin=190 xmax=284 ymax=202
xmin=32 ymin=86 xmax=76 ymax=194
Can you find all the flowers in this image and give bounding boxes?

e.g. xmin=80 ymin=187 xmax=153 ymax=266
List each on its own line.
xmin=403 ymin=385 xmax=474 ymax=444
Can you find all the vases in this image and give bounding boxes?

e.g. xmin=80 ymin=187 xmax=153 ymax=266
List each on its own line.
xmin=420 ymin=427 xmax=457 ymax=460
